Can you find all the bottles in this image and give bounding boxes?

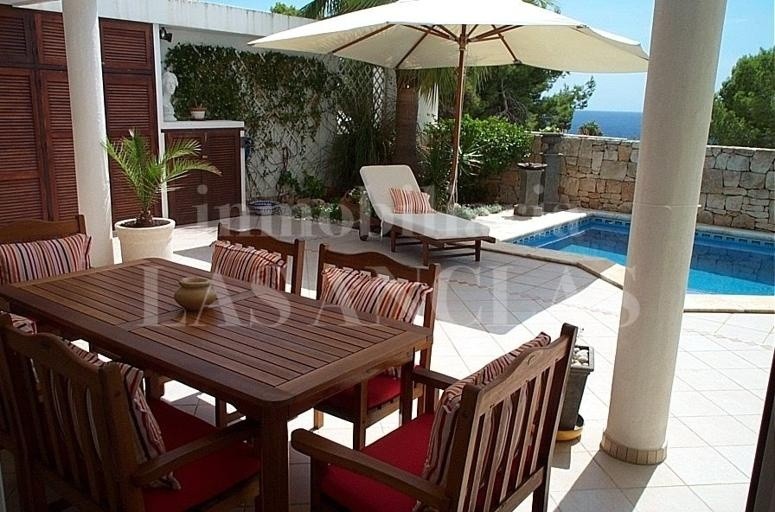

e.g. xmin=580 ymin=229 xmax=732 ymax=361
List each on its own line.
xmin=174 ymin=276 xmax=218 ymax=310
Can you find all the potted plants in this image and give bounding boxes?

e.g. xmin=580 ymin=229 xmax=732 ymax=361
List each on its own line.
xmin=186 ymin=96 xmax=209 ymax=120
xmin=99 ymin=128 xmax=223 ymax=262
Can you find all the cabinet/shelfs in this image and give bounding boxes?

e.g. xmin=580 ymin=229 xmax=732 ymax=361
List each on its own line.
xmin=1 ymin=5 xmax=162 ymax=218
xmin=162 ymin=128 xmax=242 ymax=224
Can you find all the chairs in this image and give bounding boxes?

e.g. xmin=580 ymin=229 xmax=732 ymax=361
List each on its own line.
xmin=359 ymin=163 xmax=496 ymax=267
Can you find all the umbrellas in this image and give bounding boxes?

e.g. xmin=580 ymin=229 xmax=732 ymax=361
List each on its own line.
xmin=245 ymin=1 xmax=649 ymax=205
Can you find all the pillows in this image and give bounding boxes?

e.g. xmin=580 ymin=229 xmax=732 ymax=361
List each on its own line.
xmin=389 ymin=186 xmax=436 ymax=215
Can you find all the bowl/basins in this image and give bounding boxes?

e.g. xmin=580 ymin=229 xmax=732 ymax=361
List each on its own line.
xmin=249 ymin=199 xmax=281 ymax=216
